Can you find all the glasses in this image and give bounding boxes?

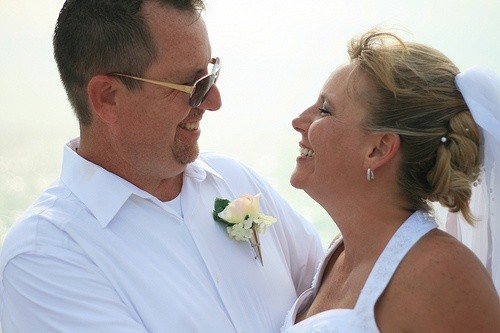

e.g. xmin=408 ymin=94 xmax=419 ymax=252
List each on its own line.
xmin=105 ymin=57 xmax=221 ymax=108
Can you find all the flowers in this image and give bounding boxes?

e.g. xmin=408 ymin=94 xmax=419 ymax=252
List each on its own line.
xmin=213 ymin=193 xmax=277 ymax=265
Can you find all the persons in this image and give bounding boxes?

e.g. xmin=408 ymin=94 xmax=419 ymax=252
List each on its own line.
xmin=0 ymin=0 xmax=324 ymax=331
xmin=279 ymin=27 xmax=500 ymax=332
